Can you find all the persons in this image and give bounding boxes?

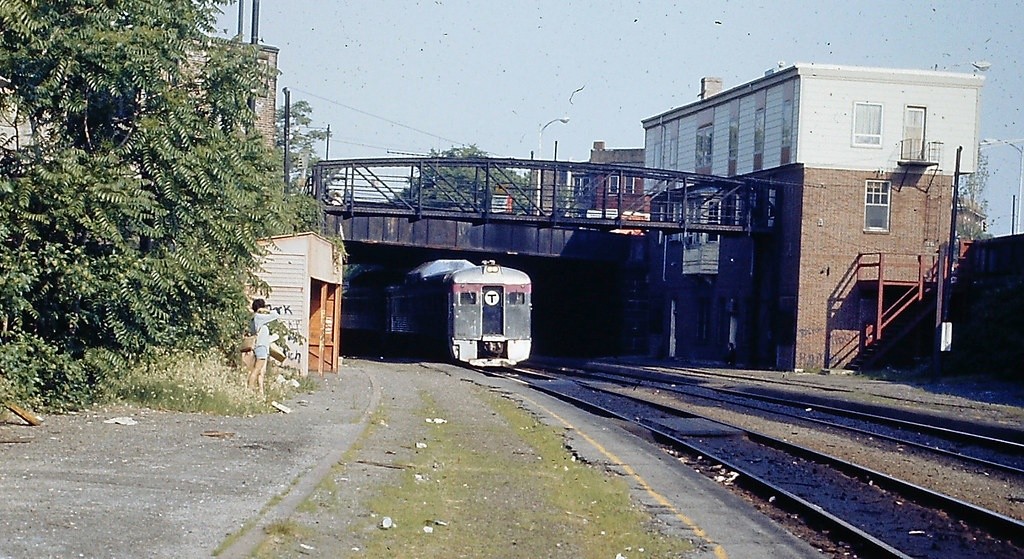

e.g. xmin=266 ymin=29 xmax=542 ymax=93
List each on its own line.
xmin=244 ymin=299 xmax=279 ymax=398
xmin=324 ymin=193 xmax=343 ymax=205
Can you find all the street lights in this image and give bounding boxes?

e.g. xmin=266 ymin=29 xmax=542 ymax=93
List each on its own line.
xmin=983 ymin=138 xmax=1023 ymax=234
xmin=537 ymin=117 xmax=570 ymax=215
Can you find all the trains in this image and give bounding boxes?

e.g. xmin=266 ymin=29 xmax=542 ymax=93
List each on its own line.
xmin=340 ymin=259 xmax=532 ymax=368
